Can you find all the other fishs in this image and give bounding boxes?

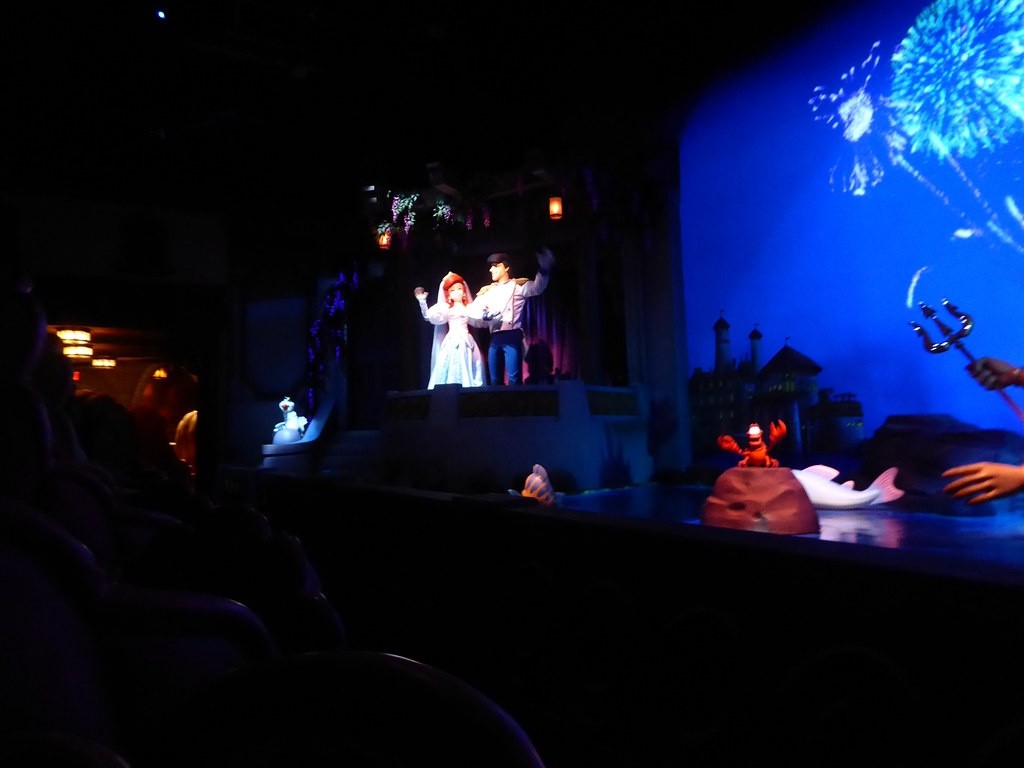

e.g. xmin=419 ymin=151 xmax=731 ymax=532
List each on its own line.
xmin=521 ymin=464 xmax=555 ymax=506
xmin=790 ymin=464 xmax=905 ymax=511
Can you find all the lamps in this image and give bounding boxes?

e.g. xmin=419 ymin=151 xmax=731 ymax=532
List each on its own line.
xmin=548 ymin=196 xmax=563 ymax=219
xmin=378 ymin=233 xmax=392 ymax=250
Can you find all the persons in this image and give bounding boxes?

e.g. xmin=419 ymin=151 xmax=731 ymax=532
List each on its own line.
xmin=415 ymin=271 xmax=486 ymax=390
xmin=941 ymin=357 xmax=1024 ymax=504
xmin=467 ymin=248 xmax=554 ymax=386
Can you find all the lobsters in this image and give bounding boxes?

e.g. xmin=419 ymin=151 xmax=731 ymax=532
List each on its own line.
xmin=717 ymin=419 xmax=786 ymax=469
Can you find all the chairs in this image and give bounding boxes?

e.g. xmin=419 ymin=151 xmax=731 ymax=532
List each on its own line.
xmin=0 ymin=471 xmax=547 ymax=767
